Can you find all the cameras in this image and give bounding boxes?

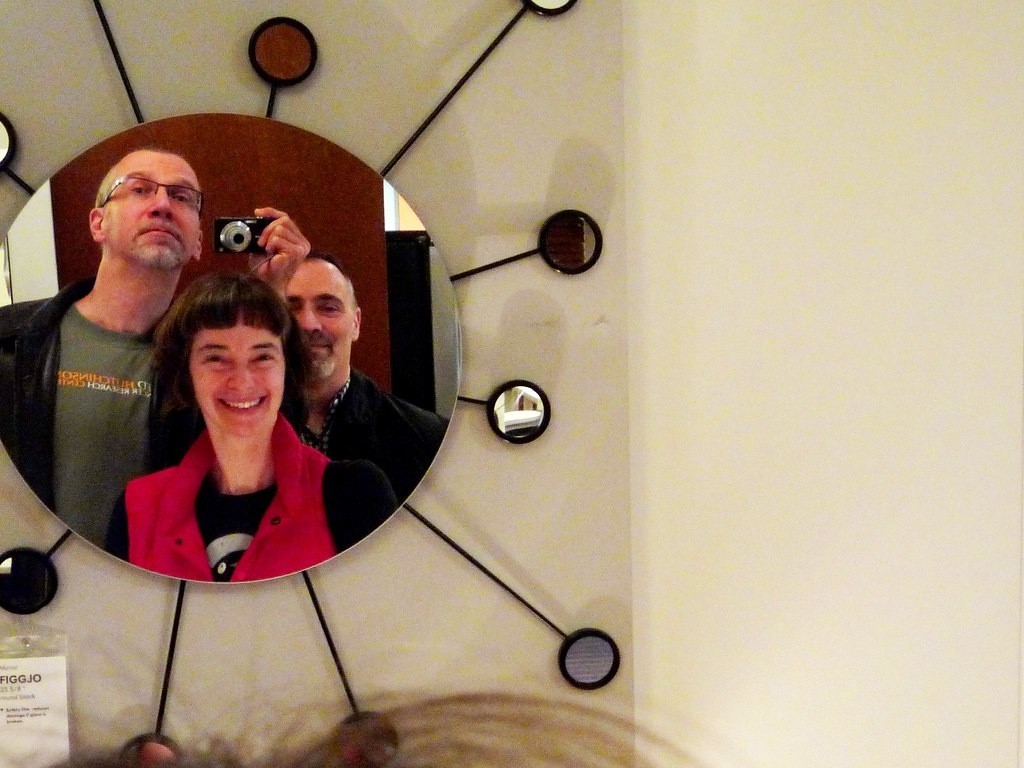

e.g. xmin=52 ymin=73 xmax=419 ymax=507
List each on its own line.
xmin=214 ymin=216 xmax=273 ymax=253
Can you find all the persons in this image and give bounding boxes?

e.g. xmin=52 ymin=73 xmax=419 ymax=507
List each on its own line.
xmin=285 ymin=250 xmax=446 ymax=506
xmin=0 ymin=148 xmax=312 ymax=558
xmin=103 ymin=274 xmax=401 ymax=591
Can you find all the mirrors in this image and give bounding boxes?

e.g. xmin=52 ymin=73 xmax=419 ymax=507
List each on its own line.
xmin=1 ymin=114 xmax=460 ymax=587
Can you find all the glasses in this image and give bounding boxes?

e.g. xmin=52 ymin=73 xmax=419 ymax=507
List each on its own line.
xmin=101 ymin=177 xmax=204 ymax=221
